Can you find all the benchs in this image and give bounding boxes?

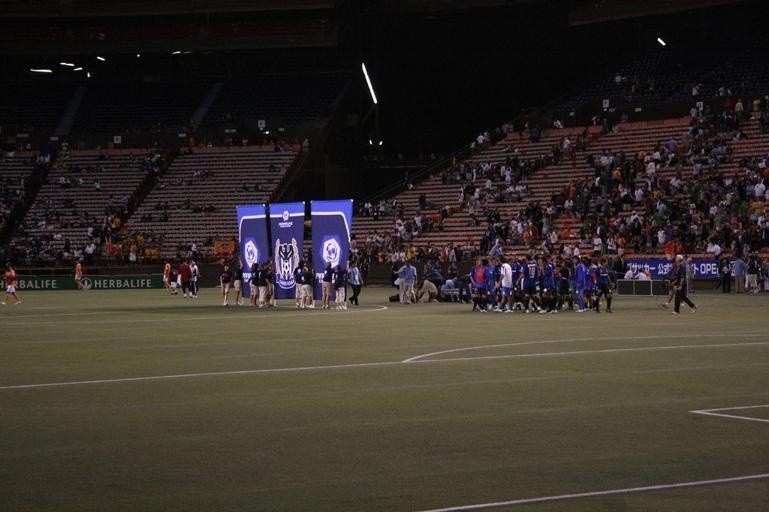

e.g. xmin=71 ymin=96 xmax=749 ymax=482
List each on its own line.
xmin=2 ymin=142 xmax=298 ymax=261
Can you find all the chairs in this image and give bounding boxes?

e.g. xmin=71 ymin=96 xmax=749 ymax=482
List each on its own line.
xmin=426 ymin=149 xmax=768 ymax=183
xmin=487 ymin=109 xmax=769 ymax=149
xmin=352 ymin=183 xmax=768 ymax=262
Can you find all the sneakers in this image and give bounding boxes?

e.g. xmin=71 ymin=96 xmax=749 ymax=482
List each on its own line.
xmin=575 ymin=308 xmax=589 ymax=312
xmin=349 ymin=297 xmax=353 ymax=304
xmin=662 ymin=301 xmax=697 ymax=315
xmin=606 ymin=309 xmax=612 ymax=312
xmin=224 ymin=301 xmax=276 ymax=308
xmin=596 ymin=310 xmax=600 ymax=312
xmin=459 ymin=299 xmax=474 ymax=304
xmin=15 ymin=302 xmax=21 ymax=305
xmin=1 ymin=302 xmax=7 ymax=305
xmin=472 ymin=307 xmax=558 ymax=314
xmin=296 ymin=303 xmax=347 ymax=309
xmin=355 ymin=302 xmax=359 ymax=305
xmin=184 ymin=295 xmax=198 ymax=300
